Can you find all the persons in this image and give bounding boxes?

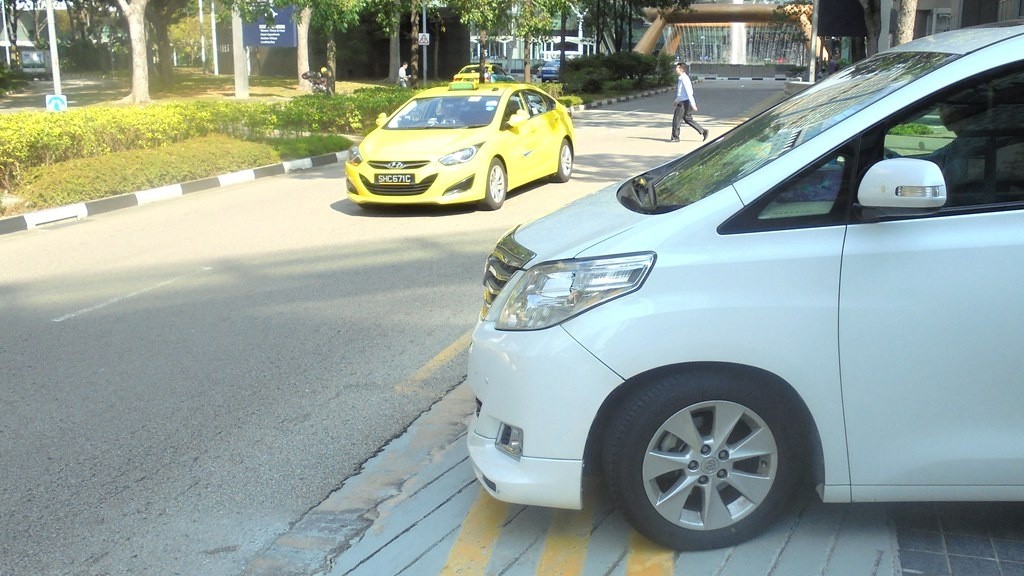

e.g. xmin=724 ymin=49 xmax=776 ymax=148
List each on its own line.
xmin=668 ymin=62 xmax=708 ymax=142
xmin=398 ymin=62 xmax=409 ymax=87
xmin=880 ymin=86 xmax=988 ymax=184
xmin=303 ymin=66 xmax=335 ymax=97
xmin=829 ymin=60 xmax=835 ymax=75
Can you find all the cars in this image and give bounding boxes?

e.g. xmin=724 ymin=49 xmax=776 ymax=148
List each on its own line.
xmin=454 ymin=64 xmax=510 ymax=83
xmin=763 ymin=48 xmax=806 ymax=66
xmin=345 ymin=81 xmax=575 ymax=212
xmin=463 ymin=27 xmax=1024 ymax=550
xmin=541 ymin=59 xmax=561 ymax=81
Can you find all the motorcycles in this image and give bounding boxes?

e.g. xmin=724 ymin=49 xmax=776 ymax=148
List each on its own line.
xmin=302 ymin=71 xmax=334 ymax=97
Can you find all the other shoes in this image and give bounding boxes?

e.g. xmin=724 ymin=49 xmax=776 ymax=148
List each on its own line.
xmin=703 ymin=129 xmax=709 ymax=141
xmin=667 ymin=139 xmax=680 ymax=142
